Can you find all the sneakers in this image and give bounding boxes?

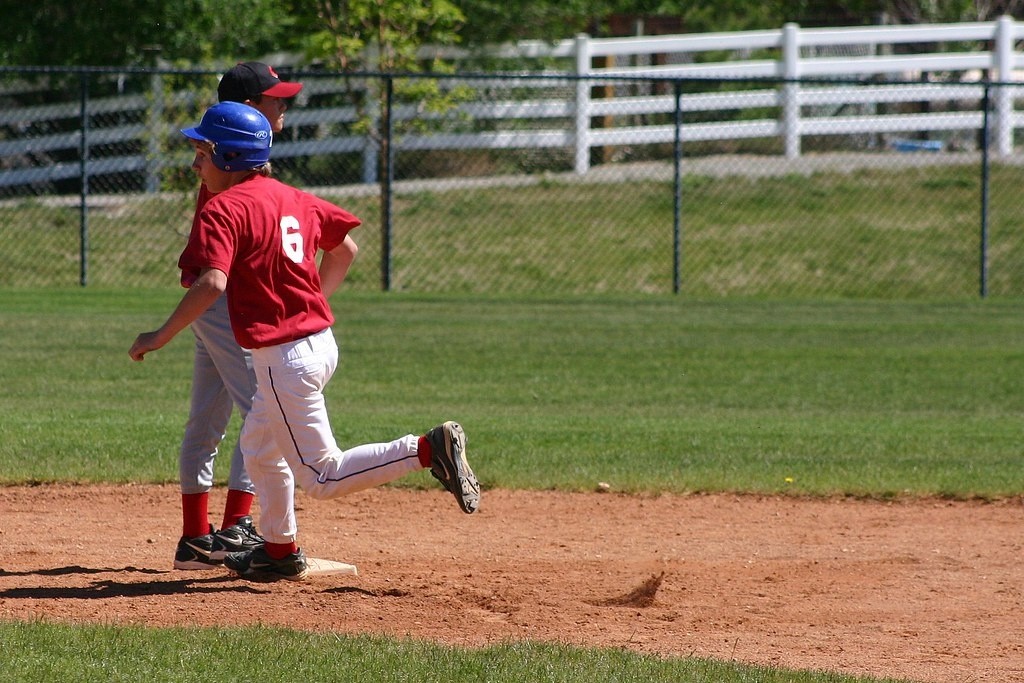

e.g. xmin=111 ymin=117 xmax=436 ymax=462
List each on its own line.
xmin=173 ymin=523 xmax=227 ymax=570
xmin=424 ymin=421 xmax=480 ymax=514
xmin=223 ymin=545 xmax=307 ymax=581
xmin=209 ymin=515 xmax=265 ymax=560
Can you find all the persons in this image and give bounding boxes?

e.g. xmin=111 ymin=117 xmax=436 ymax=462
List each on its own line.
xmin=172 ymin=61 xmax=301 ymax=569
xmin=128 ymin=101 xmax=480 ymax=578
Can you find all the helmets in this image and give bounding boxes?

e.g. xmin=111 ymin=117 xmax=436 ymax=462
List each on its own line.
xmin=179 ymin=101 xmax=272 ymax=172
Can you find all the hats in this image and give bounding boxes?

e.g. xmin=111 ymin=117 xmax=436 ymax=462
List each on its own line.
xmin=217 ymin=61 xmax=303 ymax=104
